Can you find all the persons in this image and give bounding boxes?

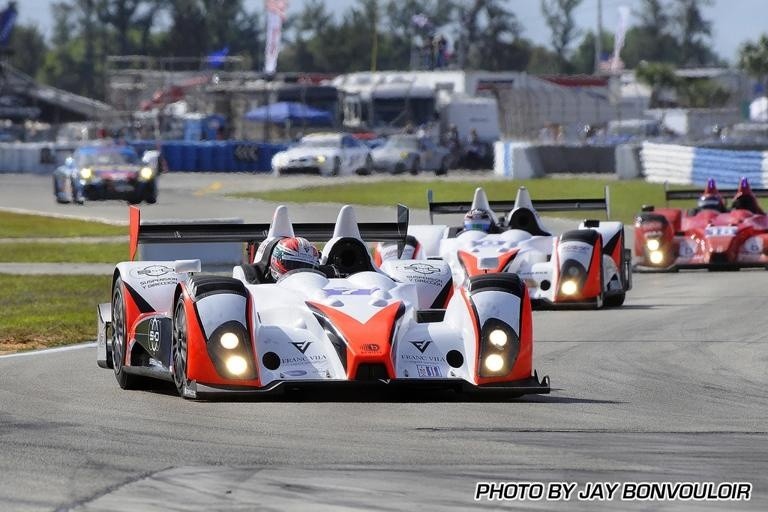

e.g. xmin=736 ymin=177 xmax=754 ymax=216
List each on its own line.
xmin=687 ymin=193 xmax=732 ymax=216
xmin=463 ymin=208 xmax=496 ymax=233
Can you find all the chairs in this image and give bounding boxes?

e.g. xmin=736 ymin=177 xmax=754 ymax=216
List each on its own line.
xmin=696 ymin=176 xmax=765 ymax=214
xmin=462 ymin=185 xmax=549 ymax=237
xmin=255 ymin=202 xmax=375 ymax=281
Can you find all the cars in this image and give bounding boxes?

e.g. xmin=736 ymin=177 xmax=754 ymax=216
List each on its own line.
xmin=375 ymin=134 xmax=450 ymax=175
xmin=270 ymin=131 xmax=372 ymax=177
xmin=97 ymin=204 xmax=549 ymax=399
xmin=53 ymin=146 xmax=158 ymax=204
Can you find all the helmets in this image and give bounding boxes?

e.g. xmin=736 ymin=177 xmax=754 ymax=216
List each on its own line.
xmin=696 ymin=194 xmax=719 ymax=208
xmin=462 ymin=208 xmax=492 ymax=231
